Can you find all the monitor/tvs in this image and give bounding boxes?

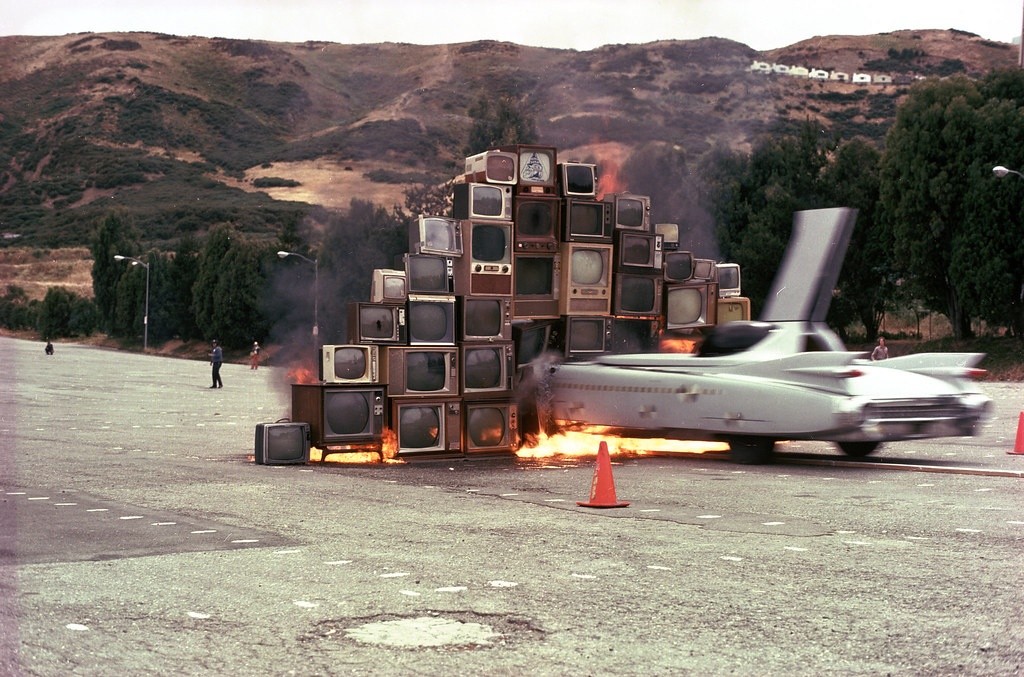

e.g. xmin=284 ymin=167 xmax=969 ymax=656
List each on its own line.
xmin=257 ymin=145 xmax=752 ymax=467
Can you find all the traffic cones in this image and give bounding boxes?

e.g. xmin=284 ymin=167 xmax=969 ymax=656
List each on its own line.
xmin=576 ymin=442 xmax=628 ymax=508
xmin=1006 ymin=411 xmax=1024 ymax=455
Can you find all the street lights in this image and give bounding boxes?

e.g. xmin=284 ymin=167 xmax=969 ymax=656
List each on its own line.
xmin=278 ymin=251 xmax=319 ymax=366
xmin=114 ymin=255 xmax=150 ymax=353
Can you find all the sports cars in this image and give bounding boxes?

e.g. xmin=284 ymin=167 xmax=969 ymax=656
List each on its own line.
xmin=517 ymin=207 xmax=1000 ymax=466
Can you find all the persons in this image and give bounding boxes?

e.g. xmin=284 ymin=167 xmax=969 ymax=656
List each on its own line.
xmin=871 ymin=336 xmax=889 ymax=360
xmin=250 ymin=341 xmax=260 ymax=370
xmin=45 ymin=341 xmax=54 ymax=355
xmin=208 ymin=339 xmax=223 ymax=388
